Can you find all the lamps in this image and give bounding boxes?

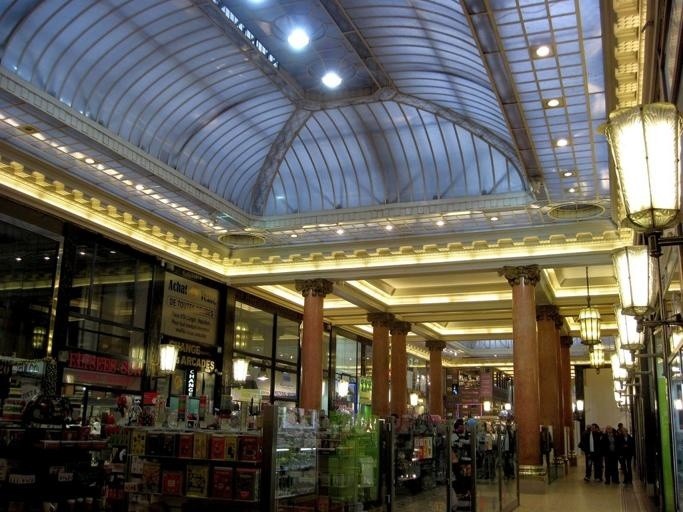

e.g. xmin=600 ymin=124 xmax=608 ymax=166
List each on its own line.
xmin=577 ymin=103 xmax=682 ymax=389
xmin=227 ymin=357 xmax=250 ymax=388
xmin=152 ymin=343 xmax=181 ymax=380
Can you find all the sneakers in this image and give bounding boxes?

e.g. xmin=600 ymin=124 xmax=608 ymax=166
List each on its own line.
xmin=583 ymin=477 xmax=633 ymax=485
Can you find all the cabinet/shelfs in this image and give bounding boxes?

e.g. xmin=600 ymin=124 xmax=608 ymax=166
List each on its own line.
xmin=24 ymin=405 xmax=382 ymax=512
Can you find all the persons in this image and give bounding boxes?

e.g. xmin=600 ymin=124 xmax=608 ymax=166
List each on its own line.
xmin=578 ymin=423 xmax=635 ymax=485
xmin=451 ymin=419 xmax=472 ymax=501
xmin=540 ymin=426 xmax=554 ymax=467
